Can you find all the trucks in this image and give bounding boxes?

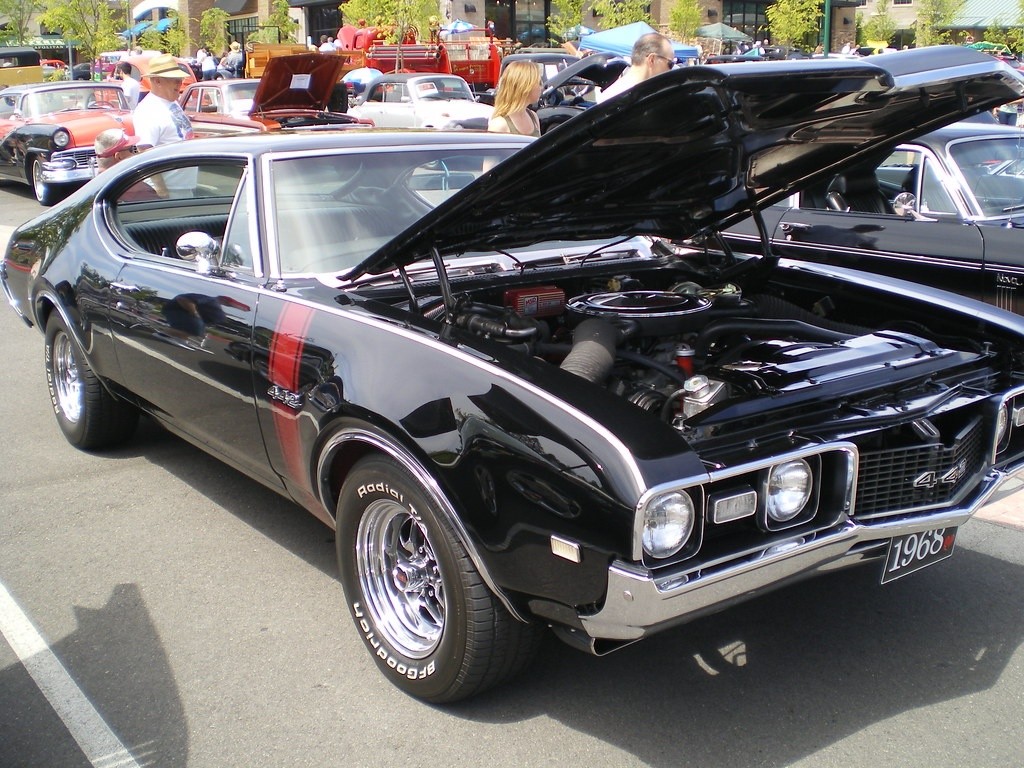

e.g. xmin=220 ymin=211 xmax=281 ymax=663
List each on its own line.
xmin=362 ymin=21 xmax=502 ymax=88
xmin=208 ymin=25 xmax=367 ymax=113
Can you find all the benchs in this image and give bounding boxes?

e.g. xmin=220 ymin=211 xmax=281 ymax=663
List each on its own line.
xmin=106 ymin=204 xmax=391 ymax=256
xmin=799 ymin=168 xmax=896 ymax=211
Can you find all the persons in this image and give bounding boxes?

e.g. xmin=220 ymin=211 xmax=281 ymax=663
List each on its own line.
xmin=309 ymin=35 xmax=342 ymax=52
xmin=95 ymin=129 xmax=161 ymax=202
xmin=598 ymin=32 xmax=674 ymax=103
xmin=194 ymin=41 xmax=242 ymax=81
xmin=902 ymin=42 xmax=909 ymax=49
xmin=814 ymin=43 xmax=824 ymax=54
xmin=133 ymin=46 xmax=143 ymax=56
xmin=991 ymin=97 xmax=1024 ymax=126
xmin=841 ymin=43 xmax=861 ymax=55
xmin=132 ymin=54 xmax=198 ymax=199
xmin=730 ymin=37 xmax=779 ymax=62
xmin=118 ymin=62 xmax=140 ymax=110
xmin=482 ymin=58 xmax=542 ymax=174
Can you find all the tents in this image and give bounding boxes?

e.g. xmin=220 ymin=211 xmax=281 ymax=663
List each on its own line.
xmin=575 ymin=21 xmax=700 ymax=68
xmin=694 ymin=20 xmax=752 ymax=56
xmin=520 ymin=26 xmax=546 ymax=45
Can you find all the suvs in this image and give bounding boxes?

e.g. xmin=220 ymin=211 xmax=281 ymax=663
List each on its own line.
xmin=979 ymin=49 xmax=1024 ymax=76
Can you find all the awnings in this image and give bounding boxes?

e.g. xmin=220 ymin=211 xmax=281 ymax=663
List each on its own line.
xmin=123 ymin=18 xmax=176 ymax=40
xmin=0 ymin=36 xmax=77 ymax=49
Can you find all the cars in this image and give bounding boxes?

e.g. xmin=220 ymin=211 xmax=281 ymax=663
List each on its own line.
xmin=697 ymin=111 xmax=1024 ymax=317
xmin=0 ymin=47 xmax=203 ymax=93
xmin=348 ymin=72 xmax=495 ymax=132
xmin=177 ymin=51 xmax=376 ymax=137
xmin=495 ymin=49 xmax=863 ymax=135
xmin=0 ymin=80 xmax=193 ymax=207
xmin=0 ymin=41 xmax=1024 ymax=707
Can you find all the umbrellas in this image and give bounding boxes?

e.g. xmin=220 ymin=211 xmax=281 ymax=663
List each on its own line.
xmin=341 ymin=66 xmax=383 ymax=84
xmin=439 ymin=18 xmax=478 ymax=41
xmin=562 ymin=23 xmax=596 ymax=43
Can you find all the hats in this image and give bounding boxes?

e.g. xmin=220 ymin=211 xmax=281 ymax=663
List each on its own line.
xmin=97 ymin=130 xmax=141 ymax=158
xmin=229 ymin=41 xmax=241 ymax=53
xmin=140 ymin=52 xmax=191 ymax=78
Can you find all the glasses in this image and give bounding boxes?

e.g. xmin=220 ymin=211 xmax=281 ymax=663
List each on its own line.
xmin=535 ymin=79 xmax=543 ymax=89
xmin=113 ymin=144 xmax=134 ymax=156
xmin=163 ymin=76 xmax=184 ymax=84
xmin=646 ymin=51 xmax=674 ymax=70
